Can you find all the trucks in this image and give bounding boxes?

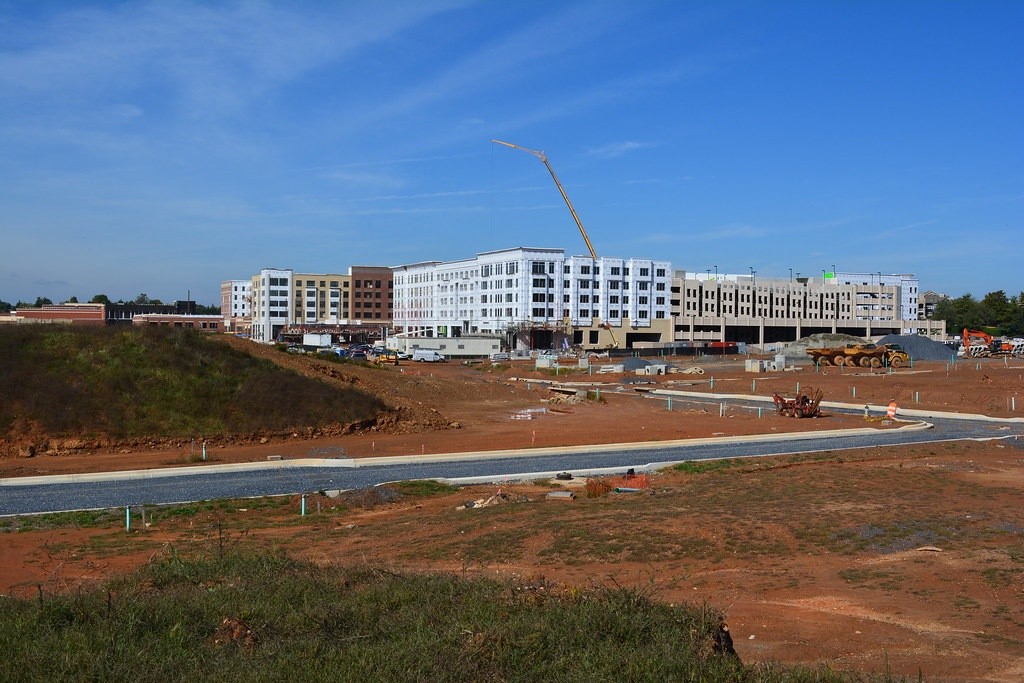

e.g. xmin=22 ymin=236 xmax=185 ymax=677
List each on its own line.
xmin=413 ymin=350 xmax=445 ymax=363
xmin=806 ymin=343 xmax=909 ymax=367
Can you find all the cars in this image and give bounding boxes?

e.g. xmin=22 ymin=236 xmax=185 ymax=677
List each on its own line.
xmin=332 ymin=344 xmax=387 ymax=363
xmin=397 ymin=351 xmax=408 ymax=360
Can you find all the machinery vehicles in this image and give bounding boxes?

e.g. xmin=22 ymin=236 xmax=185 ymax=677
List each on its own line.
xmin=961 ymin=328 xmax=1014 ymax=359
xmin=774 ymin=386 xmax=824 ymax=419
xmin=374 ymin=348 xmax=400 ymax=366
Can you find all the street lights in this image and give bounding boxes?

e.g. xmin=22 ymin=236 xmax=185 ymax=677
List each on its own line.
xmin=752 ymin=271 xmax=756 ymax=284
xmin=749 ymin=266 xmax=753 ymax=282
xmin=832 ymin=264 xmax=835 ymax=277
xmin=714 ymin=266 xmax=717 ymax=282
xmin=706 ymin=269 xmax=711 ymax=280
xmin=870 ymin=274 xmax=873 ymax=286
xmin=796 ymin=272 xmax=800 ymax=283
xmin=789 ymin=268 xmax=793 ymax=283
xmin=822 ymin=269 xmax=826 ymax=284
xmin=878 ymin=271 xmax=881 ymax=285
